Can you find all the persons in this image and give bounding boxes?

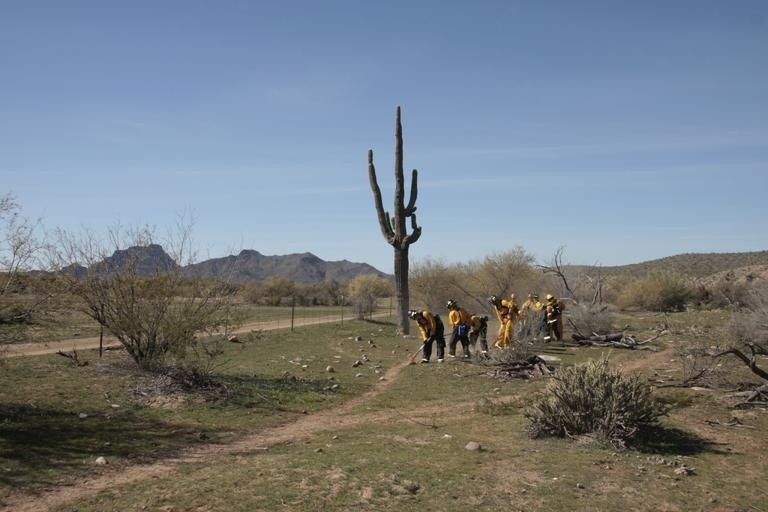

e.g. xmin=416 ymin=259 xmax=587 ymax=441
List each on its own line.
xmin=487 ymin=293 xmax=518 ymax=348
xmin=469 ymin=313 xmax=489 ymax=353
xmin=407 ymin=308 xmax=444 ymax=363
xmin=545 ymin=293 xmax=566 ymax=341
xmin=445 ymin=298 xmax=469 ymax=358
xmin=516 ymin=289 xmax=536 ymax=347
xmin=530 ymin=293 xmax=544 ymax=338
xmin=507 ymin=293 xmax=521 ymax=342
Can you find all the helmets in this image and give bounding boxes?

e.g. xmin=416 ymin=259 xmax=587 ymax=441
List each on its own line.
xmin=407 ymin=293 xmax=554 ymax=320
